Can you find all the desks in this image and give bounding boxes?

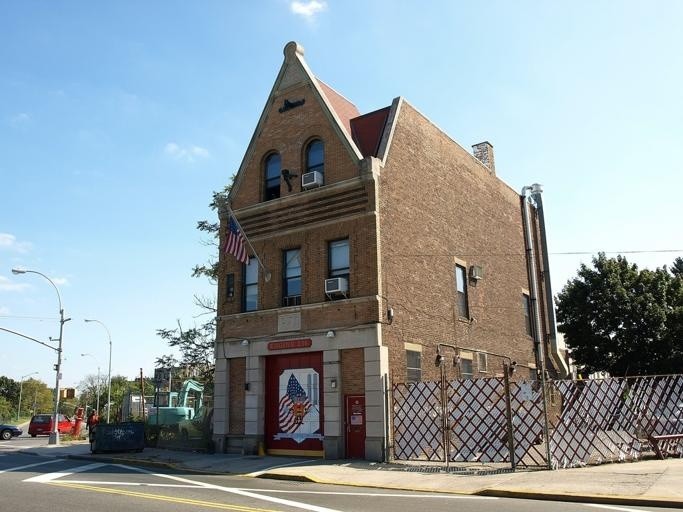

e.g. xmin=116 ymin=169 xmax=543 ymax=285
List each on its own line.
xmin=11 ymin=267 xmax=63 ymax=432
xmin=81 ymin=319 xmax=112 ymax=424
xmin=17 ymin=372 xmax=38 ymax=420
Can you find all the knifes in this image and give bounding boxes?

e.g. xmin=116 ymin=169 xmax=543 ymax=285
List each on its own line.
xmin=324 ymin=277 xmax=348 ymax=300
xmin=302 ymin=171 xmax=323 ymax=190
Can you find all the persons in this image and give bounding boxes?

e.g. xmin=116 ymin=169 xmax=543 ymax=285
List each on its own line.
xmin=86 ymin=408 xmax=101 ymax=443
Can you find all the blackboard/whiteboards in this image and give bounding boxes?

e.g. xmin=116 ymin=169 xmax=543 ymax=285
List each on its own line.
xmin=148 ymin=379 xmax=204 ymax=427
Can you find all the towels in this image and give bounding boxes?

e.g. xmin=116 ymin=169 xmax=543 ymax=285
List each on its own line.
xmin=88 ymin=421 xmax=146 ymax=454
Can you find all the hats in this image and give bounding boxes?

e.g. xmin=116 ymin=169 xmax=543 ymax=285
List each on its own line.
xmin=28 ymin=413 xmax=76 ymax=437
xmin=0 ymin=424 xmax=23 ymax=440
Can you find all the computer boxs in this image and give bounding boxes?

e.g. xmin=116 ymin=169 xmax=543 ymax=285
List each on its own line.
xmin=388 ymin=309 xmax=394 ymax=319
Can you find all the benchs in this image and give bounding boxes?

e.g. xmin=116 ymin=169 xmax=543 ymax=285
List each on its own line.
xmin=223 ymin=213 xmax=250 ymax=265
xmin=278 ymin=374 xmax=314 ymax=433
